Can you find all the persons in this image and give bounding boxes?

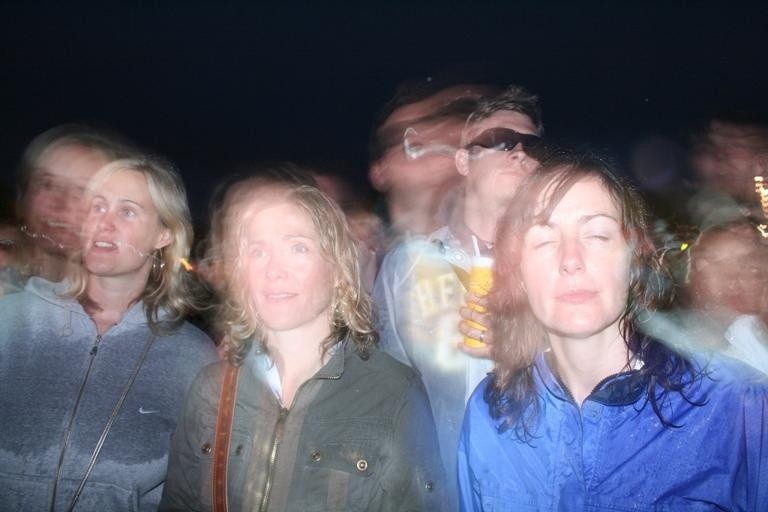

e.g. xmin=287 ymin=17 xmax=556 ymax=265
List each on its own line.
xmin=685 ymin=117 xmax=767 ymax=231
xmin=456 ymin=151 xmax=767 ymax=512
xmin=156 ymin=166 xmax=452 ymax=512
xmin=0 ymin=152 xmax=215 ymax=511
xmin=632 ymin=200 xmax=767 ymax=376
xmin=357 ymin=68 xmax=477 ymax=293
xmin=369 ymin=82 xmax=546 ymax=485
xmin=0 ymin=126 xmax=137 ymax=297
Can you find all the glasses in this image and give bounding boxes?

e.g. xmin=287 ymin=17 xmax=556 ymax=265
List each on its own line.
xmin=462 ymin=128 xmax=546 ymax=164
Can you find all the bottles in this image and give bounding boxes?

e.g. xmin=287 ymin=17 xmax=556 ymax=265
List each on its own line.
xmin=463 ymin=254 xmax=494 ymax=349
